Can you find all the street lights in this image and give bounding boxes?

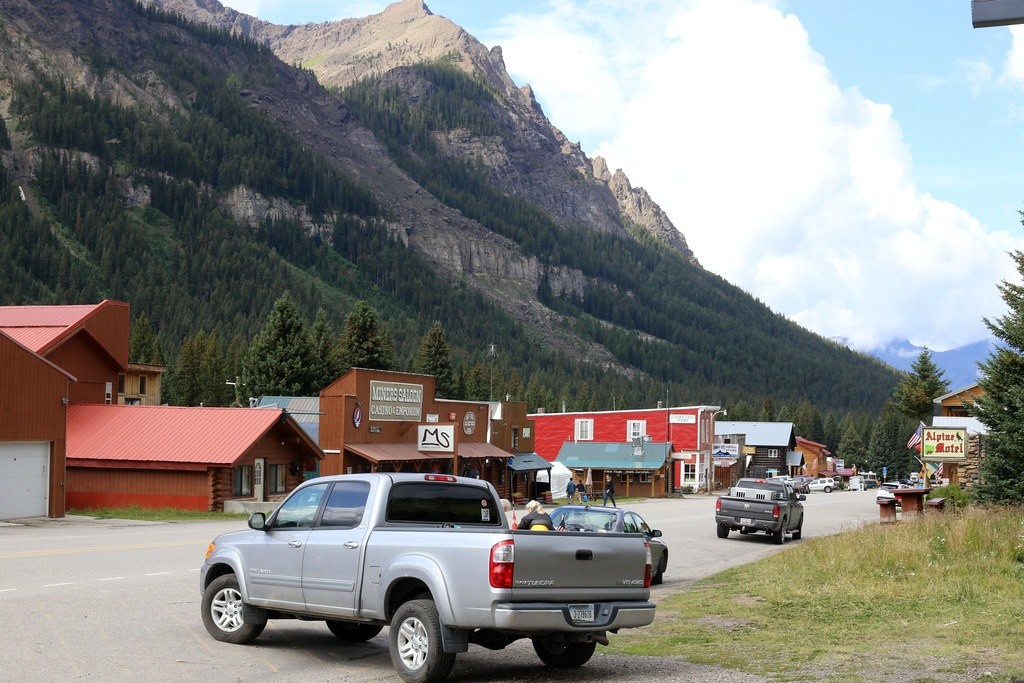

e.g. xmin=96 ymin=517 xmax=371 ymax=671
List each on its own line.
xmin=710 ymin=409 xmax=727 ymax=493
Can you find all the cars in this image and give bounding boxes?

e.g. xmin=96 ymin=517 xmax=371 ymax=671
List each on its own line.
xmin=832 ymin=472 xmax=943 ymax=509
xmin=549 ymin=503 xmax=669 ymax=586
xmin=772 ymin=474 xmax=811 ymax=495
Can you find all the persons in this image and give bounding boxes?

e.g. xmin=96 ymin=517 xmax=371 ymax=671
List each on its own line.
xmin=565 ymin=478 xmax=576 ymax=504
xmin=500 ymin=499 xmax=511 ymax=512
xmin=517 ymin=500 xmax=555 ymax=530
xmin=575 ymin=480 xmax=585 ymax=504
xmin=603 ymin=474 xmax=616 ymax=508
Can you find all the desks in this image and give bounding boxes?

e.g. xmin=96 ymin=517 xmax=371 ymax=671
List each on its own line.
xmin=889 ymin=489 xmax=931 ymax=516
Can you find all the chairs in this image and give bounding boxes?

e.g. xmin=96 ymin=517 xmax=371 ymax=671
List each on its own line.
xmin=572 ymin=512 xmax=587 ymax=528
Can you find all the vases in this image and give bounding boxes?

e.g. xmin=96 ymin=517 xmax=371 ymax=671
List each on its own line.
xmin=511 ymin=496 xmax=523 ymax=506
xmin=538 ymin=493 xmax=552 ymax=503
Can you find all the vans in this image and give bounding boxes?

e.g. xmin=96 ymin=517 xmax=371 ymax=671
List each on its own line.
xmin=808 ymin=477 xmax=835 ymax=494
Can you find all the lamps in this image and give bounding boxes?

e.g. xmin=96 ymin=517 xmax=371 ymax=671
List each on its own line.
xmin=63 ymin=398 xmax=69 ymax=405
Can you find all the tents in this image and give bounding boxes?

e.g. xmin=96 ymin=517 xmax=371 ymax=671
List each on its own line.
xmin=536 ymin=462 xmax=572 ymax=499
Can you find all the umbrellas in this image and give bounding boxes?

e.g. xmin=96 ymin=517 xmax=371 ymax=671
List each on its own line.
xmin=586 ymin=467 xmax=592 ymax=493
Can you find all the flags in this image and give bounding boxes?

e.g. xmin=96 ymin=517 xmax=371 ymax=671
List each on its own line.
xmin=557 ymin=516 xmax=569 ymax=532
xmin=802 ymin=462 xmax=808 ymax=471
xmin=511 ymin=508 xmax=518 ymax=530
xmin=812 ymin=458 xmax=818 ymax=471
xmin=907 ymin=421 xmax=943 ymax=483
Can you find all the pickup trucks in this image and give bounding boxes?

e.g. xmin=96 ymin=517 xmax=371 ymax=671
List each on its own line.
xmin=715 ymin=478 xmax=806 ymax=544
xmin=199 ymin=473 xmax=656 ymax=683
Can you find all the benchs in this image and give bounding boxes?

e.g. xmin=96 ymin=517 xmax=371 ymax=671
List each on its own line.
xmin=877 ymin=498 xmax=897 ymax=524
xmin=926 ymin=497 xmax=946 ymax=511
